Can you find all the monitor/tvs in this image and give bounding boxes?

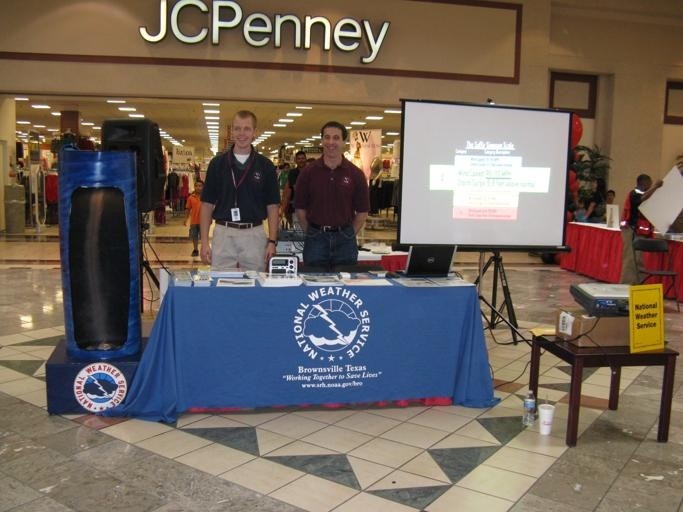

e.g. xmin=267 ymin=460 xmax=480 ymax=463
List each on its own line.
xmin=606 ymin=205 xmax=620 ymax=227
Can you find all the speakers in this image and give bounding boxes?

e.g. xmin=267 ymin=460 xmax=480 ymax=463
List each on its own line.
xmin=101 ymin=120 xmax=164 ymax=211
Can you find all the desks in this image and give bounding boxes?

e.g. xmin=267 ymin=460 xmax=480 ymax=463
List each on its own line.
xmin=559 ymin=221 xmax=683 ymax=302
xmin=528 ymin=334 xmax=680 ymax=450
xmin=90 ymin=267 xmax=502 ymax=422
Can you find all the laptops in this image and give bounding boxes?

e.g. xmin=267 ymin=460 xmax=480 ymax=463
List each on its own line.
xmin=395 ymin=244 xmax=458 ymax=277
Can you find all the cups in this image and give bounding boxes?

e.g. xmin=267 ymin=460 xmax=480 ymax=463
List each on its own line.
xmin=537 ymin=403 xmax=555 ymax=435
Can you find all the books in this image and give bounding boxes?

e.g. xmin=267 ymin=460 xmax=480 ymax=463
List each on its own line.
xmin=343 ymin=278 xmax=392 ymax=286
xmin=425 ymin=275 xmax=475 ymax=287
xmin=172 ymin=267 xmax=343 ymax=287
xmin=394 ymin=277 xmax=436 ymax=288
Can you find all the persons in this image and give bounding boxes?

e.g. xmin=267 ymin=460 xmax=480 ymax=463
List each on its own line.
xmin=572 ymin=199 xmax=595 ymax=221
xmin=181 ymin=112 xmax=371 ymax=272
xmin=618 ymin=174 xmax=662 ymax=284
xmin=597 ymin=190 xmax=615 ymax=216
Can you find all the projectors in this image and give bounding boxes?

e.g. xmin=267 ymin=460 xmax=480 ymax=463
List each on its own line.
xmin=569 ymin=282 xmax=630 ymax=317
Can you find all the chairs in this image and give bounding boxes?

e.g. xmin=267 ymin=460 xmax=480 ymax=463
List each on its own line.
xmin=630 ymin=237 xmax=681 ymax=312
xmin=380 ymin=254 xmax=408 ymax=272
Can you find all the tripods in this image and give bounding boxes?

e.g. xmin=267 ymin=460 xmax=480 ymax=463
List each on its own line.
xmin=473 ymin=255 xmax=519 ymax=345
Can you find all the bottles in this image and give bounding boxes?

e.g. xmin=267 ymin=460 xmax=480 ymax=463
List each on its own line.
xmin=522 ymin=388 xmax=535 ymax=427
xmin=68 ymin=185 xmax=131 ymax=350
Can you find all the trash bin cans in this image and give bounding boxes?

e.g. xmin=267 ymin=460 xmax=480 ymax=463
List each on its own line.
xmin=5 ymin=185 xmax=26 ymax=234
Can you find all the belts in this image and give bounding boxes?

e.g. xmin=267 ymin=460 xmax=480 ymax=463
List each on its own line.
xmin=310 ymin=224 xmax=344 ymax=232
xmin=215 ymin=220 xmax=262 ymax=228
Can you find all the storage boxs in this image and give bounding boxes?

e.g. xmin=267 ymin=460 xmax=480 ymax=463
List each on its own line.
xmin=554 ymin=307 xmax=630 ymax=348
